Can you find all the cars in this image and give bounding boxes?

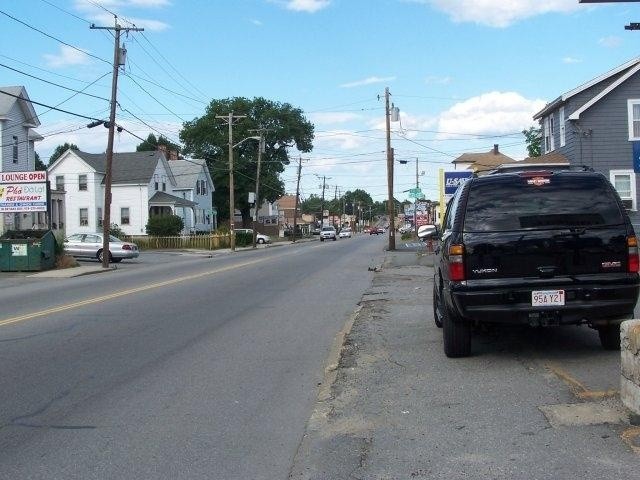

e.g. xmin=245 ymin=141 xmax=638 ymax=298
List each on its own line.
xmin=311 ymin=228 xmax=321 ymax=235
xmin=362 ymin=225 xmax=412 ymax=235
xmin=64 ymin=233 xmax=140 ymax=264
xmin=340 ymin=228 xmax=352 ymax=239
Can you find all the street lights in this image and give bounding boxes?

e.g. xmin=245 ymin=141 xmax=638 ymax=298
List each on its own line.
xmin=228 ymin=137 xmax=261 ymax=251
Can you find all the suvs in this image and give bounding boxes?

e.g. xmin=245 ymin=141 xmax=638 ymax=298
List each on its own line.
xmin=319 ymin=226 xmax=337 ymax=242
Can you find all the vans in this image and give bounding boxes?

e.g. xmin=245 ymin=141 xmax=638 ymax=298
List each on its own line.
xmin=234 ymin=229 xmax=271 ymax=244
xmin=416 ymin=160 xmax=639 ymax=358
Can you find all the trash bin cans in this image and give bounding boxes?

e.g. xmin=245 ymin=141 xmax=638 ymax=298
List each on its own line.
xmin=0 ymin=229 xmax=59 ymax=271
